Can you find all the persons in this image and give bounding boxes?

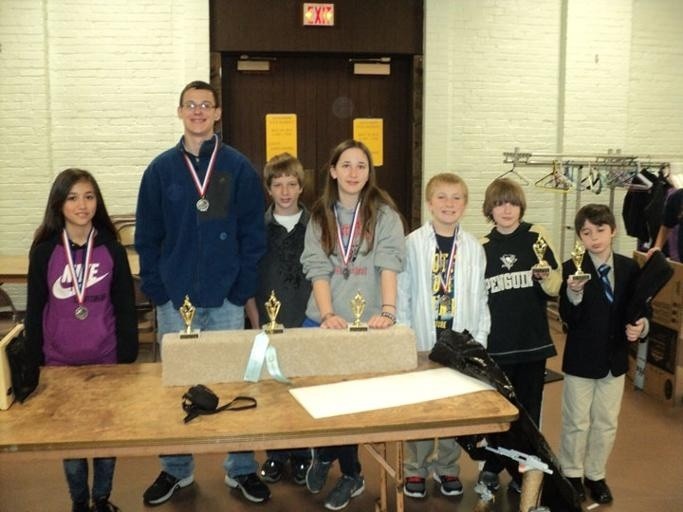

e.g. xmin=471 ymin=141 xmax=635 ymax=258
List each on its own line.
xmin=300 ymin=140 xmax=408 ymax=510
xmin=23 ymin=166 xmax=140 ymax=510
xmin=647 ymin=187 xmax=683 ymax=267
xmin=559 ymin=203 xmax=652 ymax=502
xmin=257 ymin=150 xmax=315 ymax=488
xmin=396 ymin=172 xmax=489 ymax=500
xmin=476 ymin=175 xmax=565 ymax=502
xmin=133 ymin=79 xmax=271 ymax=504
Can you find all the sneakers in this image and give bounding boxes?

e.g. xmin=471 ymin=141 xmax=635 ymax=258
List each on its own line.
xmin=71 ymin=502 xmax=90 ymax=512
xmin=223 ymin=472 xmax=271 ymax=502
xmin=142 ymin=471 xmax=193 ymax=504
xmin=323 ymin=470 xmax=364 ymax=510
xmin=403 ymin=468 xmax=522 ymax=505
xmin=304 ymin=447 xmax=331 ymax=495
xmin=93 ymin=500 xmax=114 ymax=512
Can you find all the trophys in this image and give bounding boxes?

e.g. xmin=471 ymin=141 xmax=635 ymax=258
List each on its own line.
xmin=570 ymin=238 xmax=592 ymax=280
xmin=263 ymin=288 xmax=285 ymax=334
xmin=529 ymin=231 xmax=551 ymax=273
xmin=348 ymin=289 xmax=369 ymax=332
xmin=180 ymin=293 xmax=201 ymax=339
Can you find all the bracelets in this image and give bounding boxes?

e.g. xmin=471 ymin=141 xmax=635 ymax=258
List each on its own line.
xmin=379 ymin=312 xmax=395 ymax=321
xmin=380 ymin=304 xmax=395 ymax=309
xmin=320 ymin=313 xmax=335 ymax=321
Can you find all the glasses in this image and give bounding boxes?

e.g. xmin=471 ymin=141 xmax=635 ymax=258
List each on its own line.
xmin=183 ymin=101 xmax=216 ymax=110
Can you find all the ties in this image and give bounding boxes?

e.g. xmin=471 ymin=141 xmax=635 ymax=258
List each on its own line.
xmin=598 ymin=264 xmax=614 ymax=303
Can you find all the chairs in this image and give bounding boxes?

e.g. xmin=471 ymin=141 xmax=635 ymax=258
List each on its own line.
xmin=102 ymin=213 xmax=160 ymax=359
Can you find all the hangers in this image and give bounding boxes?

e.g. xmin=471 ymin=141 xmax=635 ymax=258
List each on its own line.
xmin=534 ymin=157 xmax=676 ymax=196
xmin=490 ymin=147 xmax=531 ymax=188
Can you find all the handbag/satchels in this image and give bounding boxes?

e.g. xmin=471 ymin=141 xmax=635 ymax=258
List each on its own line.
xmin=0 ymin=284 xmax=21 ymax=338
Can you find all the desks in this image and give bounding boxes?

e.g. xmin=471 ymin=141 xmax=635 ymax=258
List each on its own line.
xmin=0 ymin=349 xmax=520 ymax=512
xmin=0 ymin=255 xmax=30 ymax=325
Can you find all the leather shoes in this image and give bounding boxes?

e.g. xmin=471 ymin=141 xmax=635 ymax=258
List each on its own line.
xmin=259 ymin=455 xmax=288 ymax=482
xmin=567 ymin=477 xmax=585 ymax=500
xmin=292 ymin=451 xmax=310 ymax=484
xmin=583 ymin=477 xmax=611 ymax=503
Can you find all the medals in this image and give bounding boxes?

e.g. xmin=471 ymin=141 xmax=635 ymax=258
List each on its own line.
xmin=438 ymin=294 xmax=451 ymax=305
xmin=193 ymin=199 xmax=211 ymax=212
xmin=341 ymin=268 xmax=349 ymax=278
xmin=73 ymin=306 xmax=89 ymax=321
